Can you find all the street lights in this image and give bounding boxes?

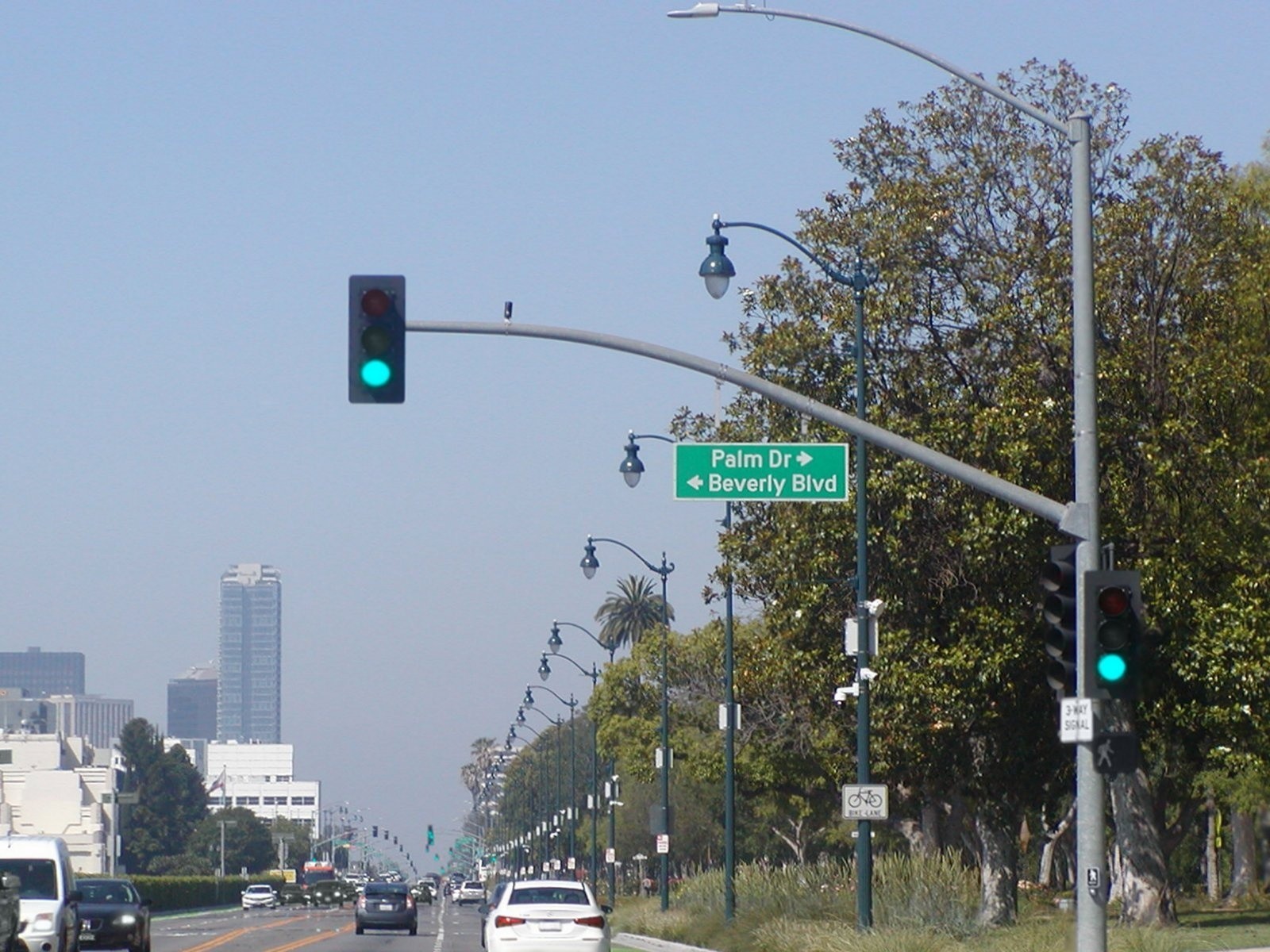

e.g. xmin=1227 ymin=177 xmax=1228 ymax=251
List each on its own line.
xmin=579 ymin=532 xmax=669 ymax=914
xmin=619 ymin=427 xmax=736 ymax=925
xmin=538 ymin=650 xmax=596 ymax=901
xmin=667 ymin=3 xmax=1108 ymax=952
xmin=548 ymin=619 xmax=615 ymax=907
xmin=697 ymin=210 xmax=878 ymax=933
xmin=523 ymin=683 xmax=577 ymax=882
xmin=478 ymin=722 xmax=550 ymax=881
xmin=329 ymin=801 xmax=350 ymax=865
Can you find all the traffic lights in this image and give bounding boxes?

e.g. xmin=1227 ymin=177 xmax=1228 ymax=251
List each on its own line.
xmin=1040 ymin=543 xmax=1078 ymax=703
xmin=428 ymin=825 xmax=434 ymax=845
xmin=349 ymin=274 xmax=406 ymax=405
xmin=1091 ymin=731 xmax=1139 ymax=774
xmin=1082 ymin=568 xmax=1147 ymax=700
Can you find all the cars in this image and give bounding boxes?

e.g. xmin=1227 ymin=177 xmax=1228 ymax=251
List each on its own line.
xmin=240 ymin=884 xmax=278 ymax=911
xmin=75 ymin=877 xmax=155 ymax=952
xmin=354 ymin=882 xmax=420 ymax=936
xmin=276 ymin=882 xmax=306 ymax=908
xmin=456 ymin=880 xmax=486 ymax=907
xmin=300 ymin=860 xmax=466 ymax=911
xmin=481 ymin=880 xmax=614 ymax=952
xmin=312 ymin=879 xmax=345 ymax=908
xmin=477 ymin=882 xmax=538 ymax=948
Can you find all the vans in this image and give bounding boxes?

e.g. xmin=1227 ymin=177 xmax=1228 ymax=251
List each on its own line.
xmin=0 ymin=834 xmax=75 ymax=952
